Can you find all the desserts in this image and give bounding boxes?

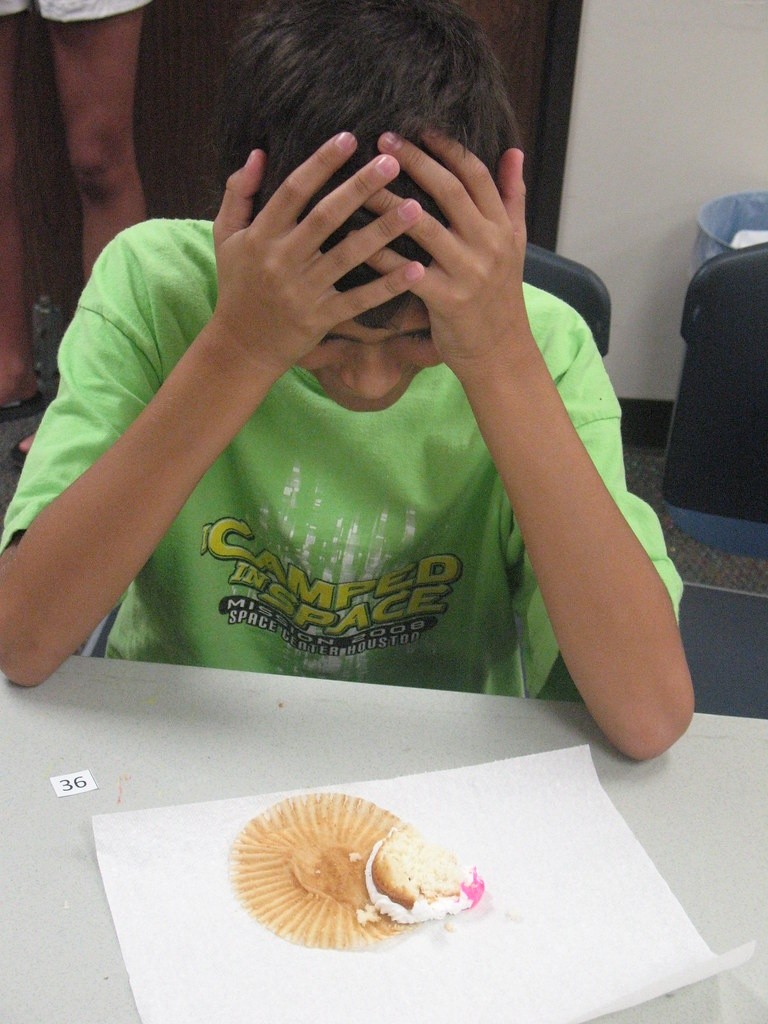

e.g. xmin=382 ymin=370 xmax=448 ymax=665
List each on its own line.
xmin=365 ymin=828 xmax=484 ymax=925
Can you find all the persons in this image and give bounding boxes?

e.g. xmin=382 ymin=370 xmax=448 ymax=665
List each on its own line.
xmin=0 ymin=1 xmax=695 ymax=763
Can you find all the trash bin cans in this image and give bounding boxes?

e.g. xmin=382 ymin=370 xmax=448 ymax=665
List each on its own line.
xmin=690 ymin=190 xmax=768 ymax=281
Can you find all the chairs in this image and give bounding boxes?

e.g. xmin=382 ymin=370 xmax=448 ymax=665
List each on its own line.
xmin=662 ymin=242 xmax=768 ymax=560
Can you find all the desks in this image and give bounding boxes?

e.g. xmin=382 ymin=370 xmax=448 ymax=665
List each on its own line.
xmin=0 ymin=653 xmax=768 ymax=1024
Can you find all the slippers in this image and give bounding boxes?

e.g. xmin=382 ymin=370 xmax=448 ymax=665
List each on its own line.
xmin=10 ymin=431 xmax=36 ymax=468
xmin=0 ymin=388 xmax=42 ymax=423
